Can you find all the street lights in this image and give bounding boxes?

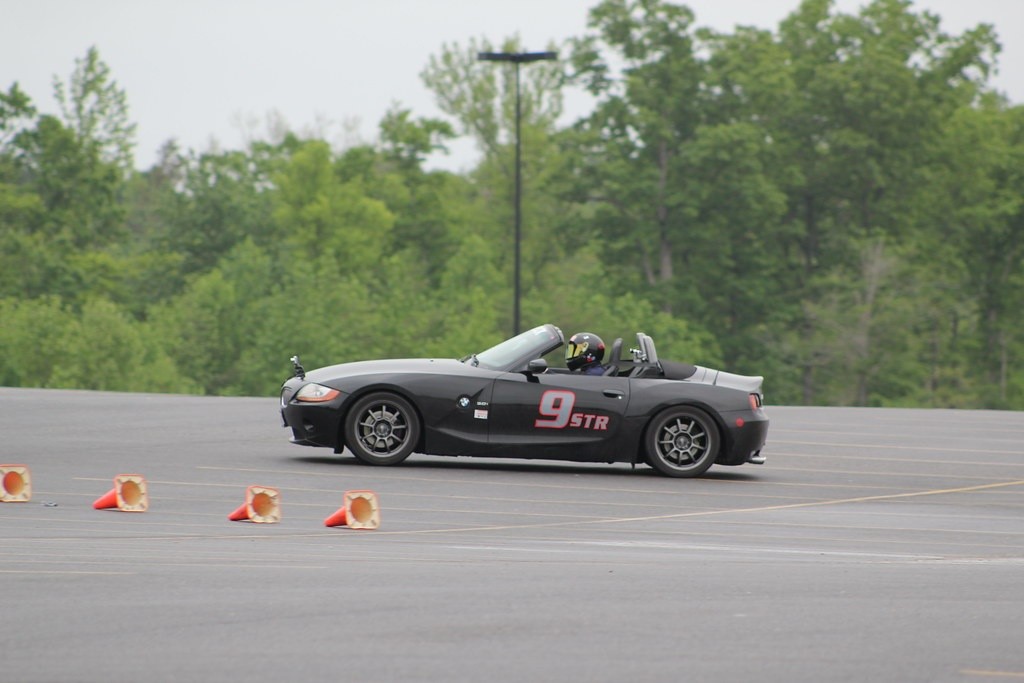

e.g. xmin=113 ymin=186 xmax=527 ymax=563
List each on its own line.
xmin=477 ymin=47 xmax=557 ymax=340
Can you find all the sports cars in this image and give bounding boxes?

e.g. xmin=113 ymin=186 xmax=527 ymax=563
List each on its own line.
xmin=280 ymin=322 xmax=773 ymax=476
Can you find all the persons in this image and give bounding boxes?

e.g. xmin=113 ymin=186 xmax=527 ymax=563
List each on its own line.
xmin=543 ymin=333 xmax=606 ymax=376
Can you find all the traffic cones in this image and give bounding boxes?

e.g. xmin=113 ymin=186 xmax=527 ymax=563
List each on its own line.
xmin=93 ymin=472 xmax=148 ymax=513
xmin=324 ymin=489 xmax=381 ymax=531
xmin=0 ymin=464 xmax=32 ymax=503
xmin=226 ymin=485 xmax=282 ymax=524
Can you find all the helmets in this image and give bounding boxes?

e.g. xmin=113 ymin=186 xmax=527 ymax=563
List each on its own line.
xmin=564 ymin=332 xmax=605 ymax=371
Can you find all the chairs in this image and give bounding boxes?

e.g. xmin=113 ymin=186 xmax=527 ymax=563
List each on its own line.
xmin=635 ymin=336 xmax=659 ymax=378
xmin=630 ymin=331 xmax=646 ymax=377
xmin=601 ymin=338 xmax=624 ymax=377
